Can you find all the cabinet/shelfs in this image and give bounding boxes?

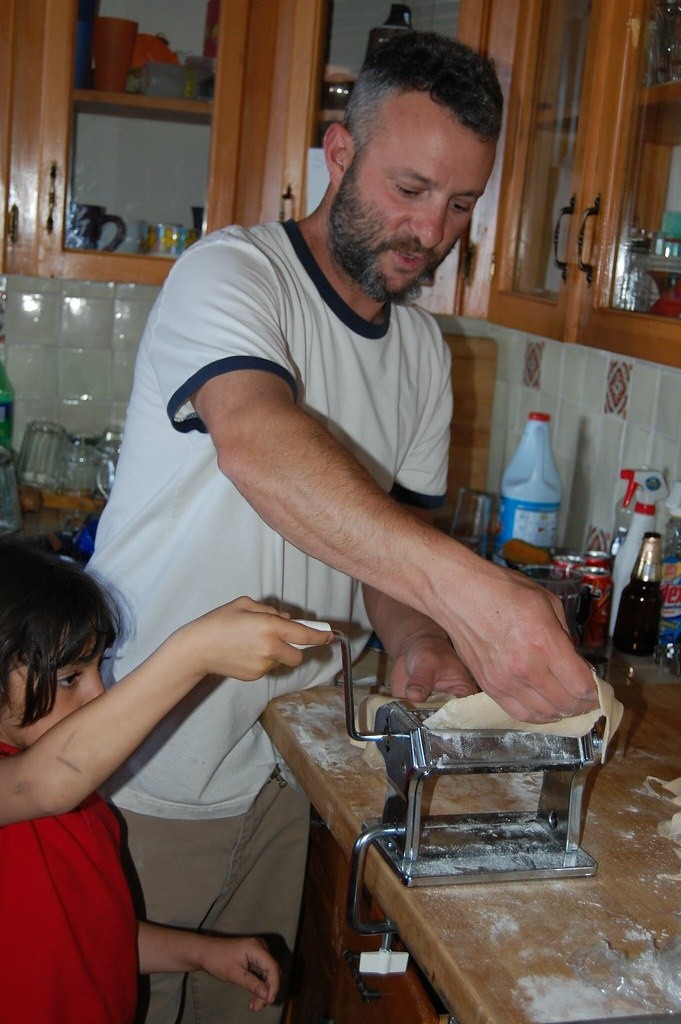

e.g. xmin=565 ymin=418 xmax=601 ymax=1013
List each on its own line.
xmin=279 ymin=0 xmax=490 ymax=316
xmin=33 ymin=0 xmax=250 ymax=287
xmin=486 ymin=0 xmax=681 ymax=368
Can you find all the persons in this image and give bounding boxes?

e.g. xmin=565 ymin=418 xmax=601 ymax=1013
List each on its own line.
xmin=83 ymin=33 xmax=603 ymax=1024
xmin=1 ymin=544 xmax=333 ymax=1023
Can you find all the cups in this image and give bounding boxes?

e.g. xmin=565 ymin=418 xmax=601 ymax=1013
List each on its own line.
xmin=93 ymin=16 xmax=139 ymax=93
xmin=67 ymin=430 xmax=124 ymax=501
xmin=17 ymin=419 xmax=67 ymax=492
xmin=0 ymin=445 xmax=23 ymax=535
xmin=64 ymin=203 xmax=127 ymax=252
xmin=520 ymin=567 xmax=582 ymax=658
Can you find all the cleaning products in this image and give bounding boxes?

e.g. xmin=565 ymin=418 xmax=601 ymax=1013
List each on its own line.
xmin=607 ymin=468 xmax=681 ymax=647
xmin=488 ymin=410 xmax=564 ymax=572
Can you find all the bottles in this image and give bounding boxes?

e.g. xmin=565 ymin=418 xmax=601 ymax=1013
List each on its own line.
xmin=615 ymin=531 xmax=665 ymax=657
xmin=0 ymin=334 xmax=16 ymax=452
xmin=491 ymin=412 xmax=564 ymax=567
